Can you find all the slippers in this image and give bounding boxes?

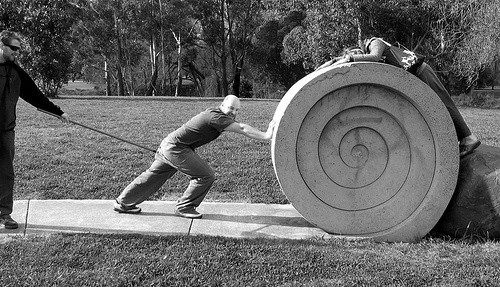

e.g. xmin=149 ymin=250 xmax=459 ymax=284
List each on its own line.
xmin=460 ymin=140 xmax=481 ymax=158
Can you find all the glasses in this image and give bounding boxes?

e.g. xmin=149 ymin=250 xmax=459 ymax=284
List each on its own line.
xmin=4 ymin=44 xmax=21 ymax=51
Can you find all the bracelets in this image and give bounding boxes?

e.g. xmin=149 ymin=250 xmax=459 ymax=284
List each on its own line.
xmin=348 ymin=55 xmax=354 ymax=62
xmin=330 ymin=58 xmax=337 ymax=64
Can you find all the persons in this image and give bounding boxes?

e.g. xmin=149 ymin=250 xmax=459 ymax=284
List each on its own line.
xmin=113 ymin=95 xmax=275 ymax=218
xmin=314 ymin=36 xmax=481 ymax=156
xmin=0 ymin=30 xmax=71 ymax=229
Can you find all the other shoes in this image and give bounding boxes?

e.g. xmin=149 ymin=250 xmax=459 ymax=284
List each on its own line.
xmin=0 ymin=215 xmax=18 ymax=230
xmin=114 ymin=199 xmax=142 ymax=213
xmin=176 ymin=208 xmax=202 ymax=218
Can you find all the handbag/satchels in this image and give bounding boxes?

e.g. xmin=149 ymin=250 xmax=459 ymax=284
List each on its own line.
xmin=366 ymin=38 xmax=425 ymax=72
